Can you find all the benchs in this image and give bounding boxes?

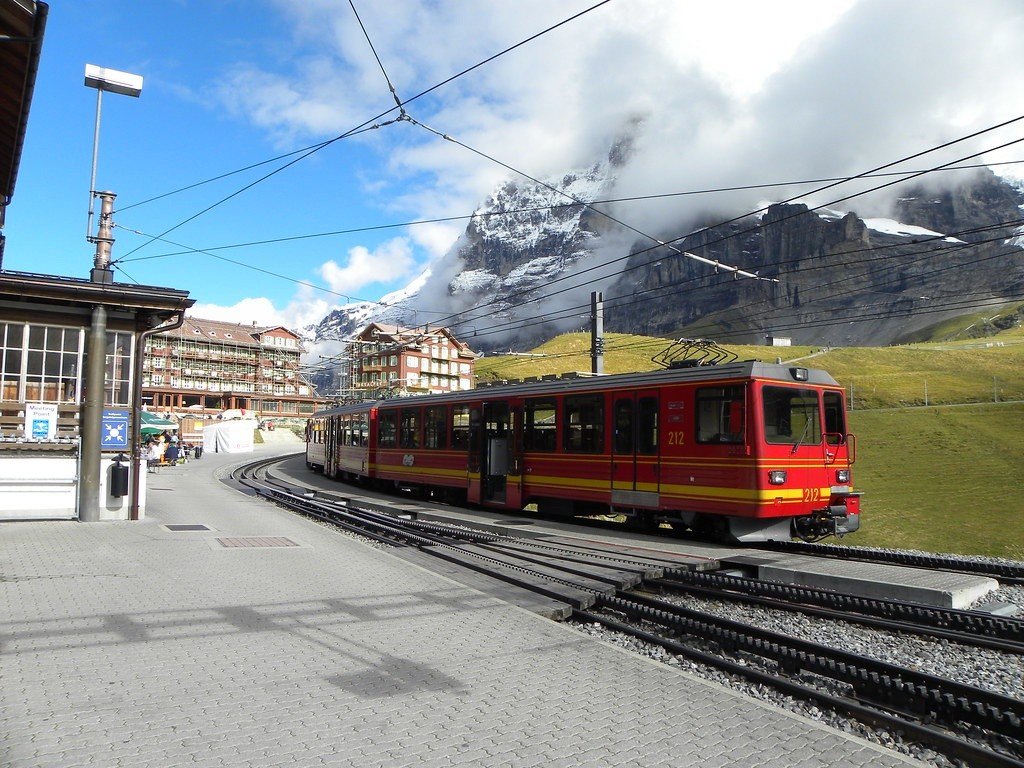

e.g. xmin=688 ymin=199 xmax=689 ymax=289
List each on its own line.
xmin=152 ymin=463 xmax=169 ymax=473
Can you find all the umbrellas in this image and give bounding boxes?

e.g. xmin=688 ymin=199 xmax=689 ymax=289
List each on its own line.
xmin=140 ymin=411 xmax=179 ymax=430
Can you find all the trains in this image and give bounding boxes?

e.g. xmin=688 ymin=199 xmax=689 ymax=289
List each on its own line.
xmin=305 ymin=361 xmax=864 ymax=547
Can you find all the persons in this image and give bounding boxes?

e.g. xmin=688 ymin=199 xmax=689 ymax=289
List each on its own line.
xmin=144 ymin=431 xmax=179 ymax=472
xmin=207 ymin=412 xmax=213 ymax=419
xmin=163 ymin=411 xmax=170 ymax=420
xmin=217 ymin=411 xmax=223 ymax=420
xmin=261 ymin=422 xmax=265 ymax=430
xmin=268 ymin=420 xmax=272 ymax=429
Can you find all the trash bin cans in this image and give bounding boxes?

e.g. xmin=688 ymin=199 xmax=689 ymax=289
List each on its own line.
xmin=195 ymin=446 xmax=202 ymax=459
xmin=111 ymin=452 xmax=129 ymax=495
